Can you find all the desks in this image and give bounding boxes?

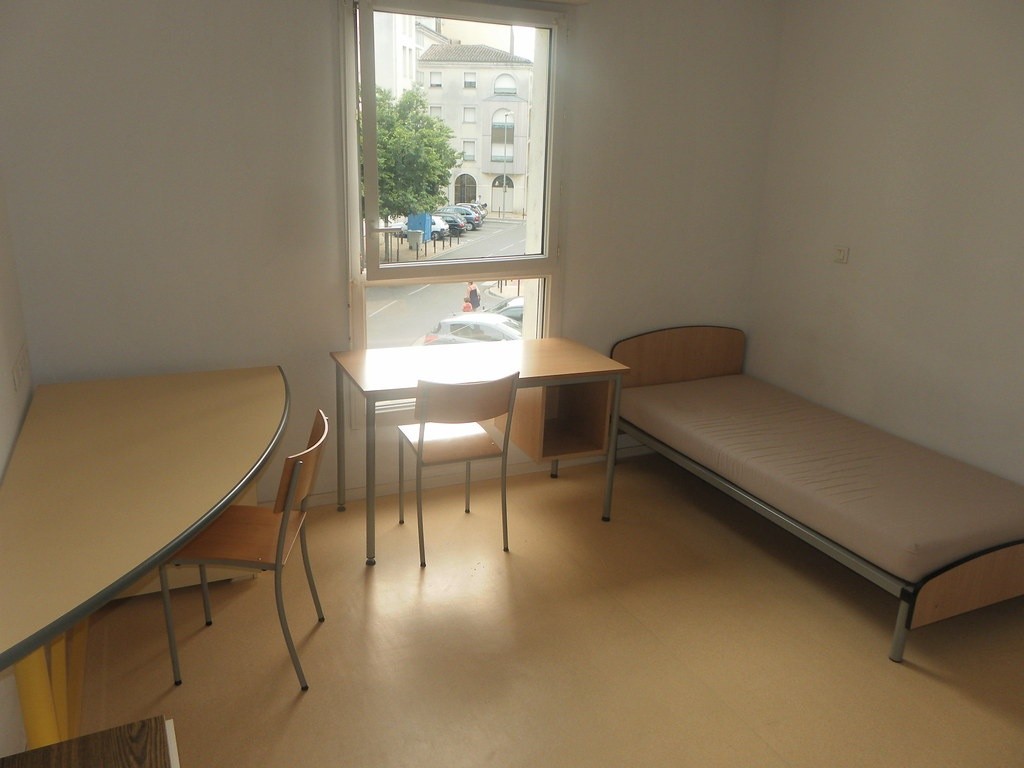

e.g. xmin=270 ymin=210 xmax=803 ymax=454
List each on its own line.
xmin=330 ymin=336 xmax=634 ymax=566
xmin=0 ymin=364 xmax=293 ymax=752
xmin=0 ymin=714 xmax=170 ymax=768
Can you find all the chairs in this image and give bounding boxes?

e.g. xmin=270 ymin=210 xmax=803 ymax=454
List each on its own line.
xmin=157 ymin=407 xmax=329 ymax=695
xmin=396 ymin=370 xmax=522 ymax=568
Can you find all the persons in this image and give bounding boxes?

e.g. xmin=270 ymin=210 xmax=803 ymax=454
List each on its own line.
xmin=467 ymin=281 xmax=481 ymax=312
xmin=462 ymin=298 xmax=473 ymax=312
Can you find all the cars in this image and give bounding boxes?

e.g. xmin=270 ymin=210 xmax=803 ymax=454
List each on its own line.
xmin=433 ymin=212 xmax=467 ymax=237
xmin=436 ymin=205 xmax=483 ymax=231
xmin=483 ymin=296 xmax=523 ymax=322
xmin=387 ymin=216 xmax=450 ymax=241
xmin=423 ymin=312 xmax=522 ymax=346
xmin=456 ymin=203 xmax=487 ymax=219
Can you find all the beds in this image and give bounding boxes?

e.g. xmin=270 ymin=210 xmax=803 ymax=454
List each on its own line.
xmin=608 ymin=324 xmax=1024 ymax=665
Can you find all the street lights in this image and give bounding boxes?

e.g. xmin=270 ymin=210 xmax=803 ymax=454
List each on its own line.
xmin=502 ymin=111 xmax=515 ymax=220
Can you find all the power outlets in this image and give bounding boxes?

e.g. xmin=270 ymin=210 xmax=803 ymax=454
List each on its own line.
xmin=12 ymin=357 xmax=30 ymax=393
